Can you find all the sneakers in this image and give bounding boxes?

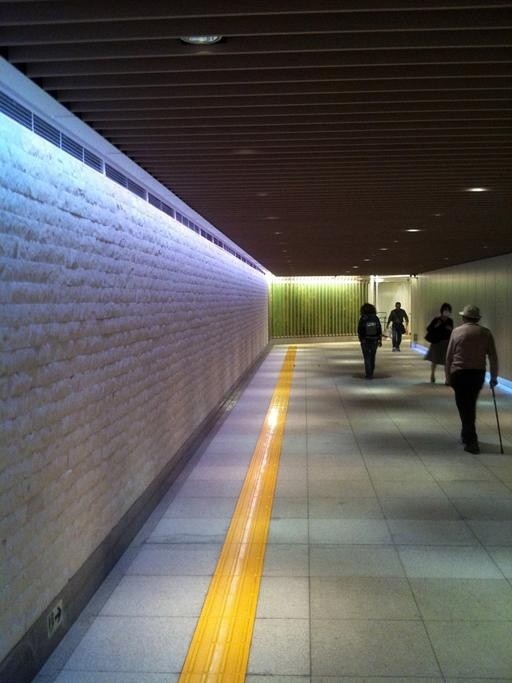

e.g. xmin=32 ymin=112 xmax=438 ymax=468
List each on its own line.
xmin=390 ymin=346 xmax=401 ymax=352
xmin=462 ymin=442 xmax=481 ymax=455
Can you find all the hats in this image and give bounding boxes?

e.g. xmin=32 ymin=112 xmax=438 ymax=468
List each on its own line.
xmin=458 ymin=305 xmax=483 ymax=320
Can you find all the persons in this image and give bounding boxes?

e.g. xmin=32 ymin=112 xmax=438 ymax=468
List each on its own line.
xmin=427 ymin=302 xmax=454 ymax=386
xmin=386 ymin=302 xmax=409 ymax=353
xmin=445 ymin=305 xmax=499 ymax=455
xmin=357 ymin=303 xmax=382 ymax=380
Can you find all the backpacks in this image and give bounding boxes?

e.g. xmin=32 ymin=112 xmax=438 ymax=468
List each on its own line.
xmin=361 ymin=318 xmax=379 ymax=340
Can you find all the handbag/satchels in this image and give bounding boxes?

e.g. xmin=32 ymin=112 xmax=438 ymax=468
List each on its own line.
xmin=395 ymin=322 xmax=406 ymax=334
